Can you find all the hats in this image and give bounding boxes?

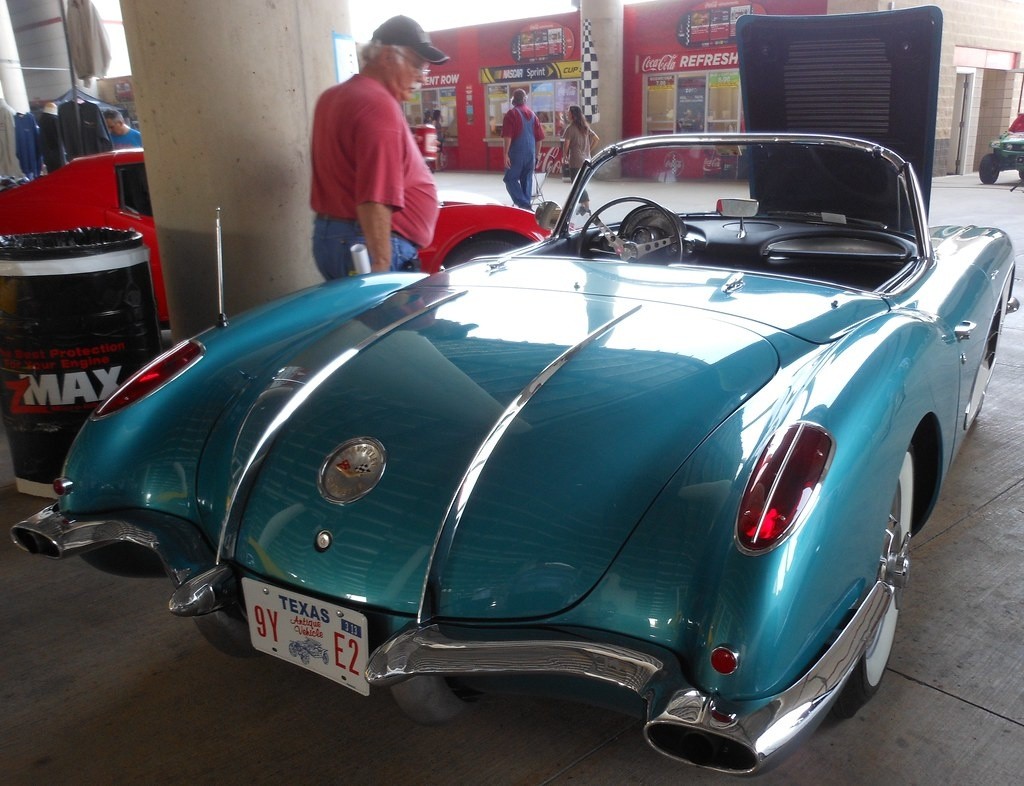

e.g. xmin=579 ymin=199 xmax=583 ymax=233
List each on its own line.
xmin=514 ymin=89 xmax=526 ymax=99
xmin=371 ymin=16 xmax=451 ymax=65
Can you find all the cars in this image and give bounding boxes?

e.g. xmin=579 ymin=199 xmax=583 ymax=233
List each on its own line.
xmin=978 ymin=113 xmax=1024 ymax=184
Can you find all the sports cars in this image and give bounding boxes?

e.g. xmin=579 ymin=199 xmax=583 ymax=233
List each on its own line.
xmin=11 ymin=7 xmax=1020 ymax=776
xmin=0 ymin=144 xmax=548 ymax=330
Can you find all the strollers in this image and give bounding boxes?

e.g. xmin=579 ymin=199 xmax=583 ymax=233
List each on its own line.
xmin=511 ymin=155 xmax=554 ymax=209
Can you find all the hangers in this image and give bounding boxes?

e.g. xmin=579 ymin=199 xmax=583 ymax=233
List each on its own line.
xmin=72 ymin=95 xmax=85 ymax=104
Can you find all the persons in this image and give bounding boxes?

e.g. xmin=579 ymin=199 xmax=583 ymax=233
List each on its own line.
xmin=104 ymin=109 xmax=142 ymax=150
xmin=423 ymin=108 xmax=445 ymax=171
xmin=500 ymin=89 xmax=546 ymax=212
xmin=562 ymin=105 xmax=601 ymax=216
xmin=311 ymin=14 xmax=450 ymax=282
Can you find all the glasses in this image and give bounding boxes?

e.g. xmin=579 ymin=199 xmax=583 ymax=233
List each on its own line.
xmin=391 ymin=49 xmax=431 ymax=76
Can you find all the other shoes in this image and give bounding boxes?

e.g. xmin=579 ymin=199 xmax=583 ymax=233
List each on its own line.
xmin=576 ymin=207 xmax=591 ymax=217
